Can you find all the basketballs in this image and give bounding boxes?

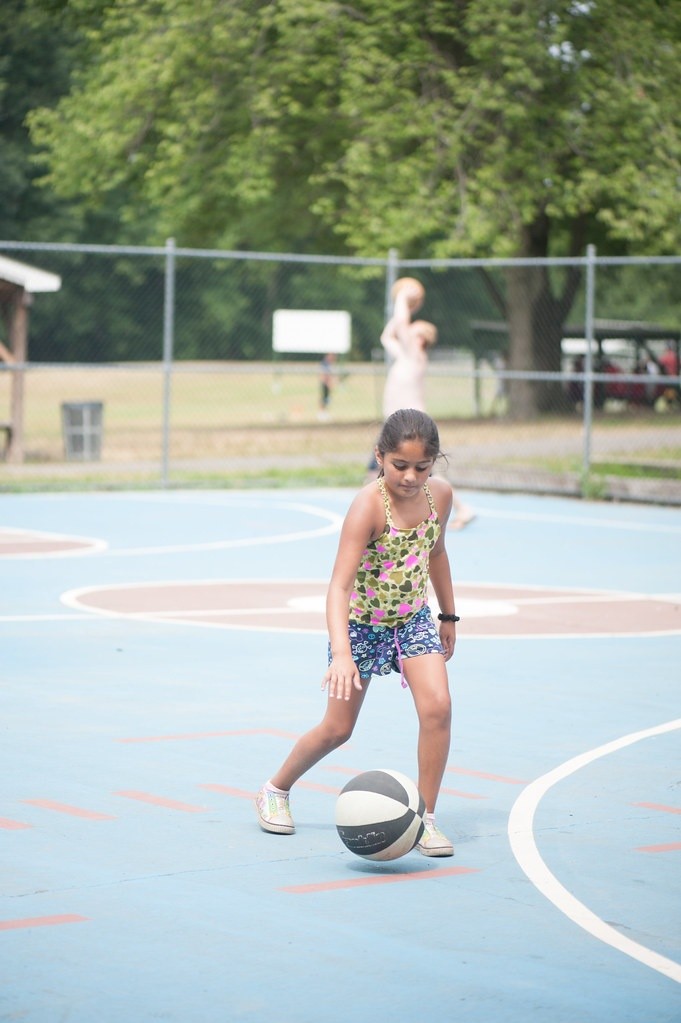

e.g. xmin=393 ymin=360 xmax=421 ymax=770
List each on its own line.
xmin=333 ymin=766 xmax=427 ymax=862
xmin=389 ymin=276 xmax=425 ymax=314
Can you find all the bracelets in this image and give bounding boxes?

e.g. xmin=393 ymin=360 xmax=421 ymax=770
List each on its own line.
xmin=438 ymin=613 xmax=460 ymax=623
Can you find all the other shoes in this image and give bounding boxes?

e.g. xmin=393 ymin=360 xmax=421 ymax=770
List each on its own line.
xmin=448 ymin=507 xmax=474 ymax=529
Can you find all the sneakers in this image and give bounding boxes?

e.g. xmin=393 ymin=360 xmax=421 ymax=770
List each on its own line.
xmin=413 ymin=825 xmax=454 ymax=857
xmin=254 ymin=787 xmax=295 ymax=834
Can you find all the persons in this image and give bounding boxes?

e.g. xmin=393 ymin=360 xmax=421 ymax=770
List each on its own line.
xmin=319 ymin=352 xmax=338 ymax=423
xmin=490 ymin=349 xmax=512 ymax=417
xmin=255 ymin=409 xmax=456 ymax=860
xmin=366 ymin=286 xmax=438 ymax=485
